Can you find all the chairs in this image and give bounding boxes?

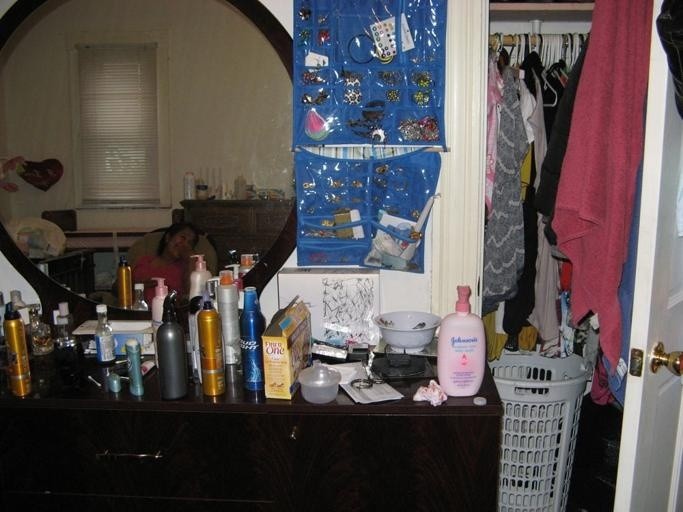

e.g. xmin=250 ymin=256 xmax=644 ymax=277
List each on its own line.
xmin=86 ymin=227 xmax=219 ymax=307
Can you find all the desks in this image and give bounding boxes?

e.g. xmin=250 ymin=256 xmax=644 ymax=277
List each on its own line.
xmin=0 ymin=340 xmax=504 ymax=511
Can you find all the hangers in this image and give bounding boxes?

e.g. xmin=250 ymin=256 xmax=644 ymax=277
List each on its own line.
xmin=493 ymin=32 xmax=588 ymax=108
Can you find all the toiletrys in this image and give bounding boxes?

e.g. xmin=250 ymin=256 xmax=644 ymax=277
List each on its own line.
xmin=56 ymin=301 xmax=75 ymax=350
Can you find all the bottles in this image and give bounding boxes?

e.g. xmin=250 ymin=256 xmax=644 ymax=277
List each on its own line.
xmin=178 ymin=170 xmax=248 ymax=203
xmin=115 ymin=251 xmax=258 ymax=314
xmin=0 ymin=270 xmax=263 ymax=402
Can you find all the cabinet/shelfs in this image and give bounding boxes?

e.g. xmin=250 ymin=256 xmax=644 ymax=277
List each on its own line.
xmin=179 ymin=195 xmax=296 ymax=276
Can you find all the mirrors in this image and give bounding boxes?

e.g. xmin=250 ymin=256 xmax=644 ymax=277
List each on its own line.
xmin=0 ymin=0 xmax=297 ymax=339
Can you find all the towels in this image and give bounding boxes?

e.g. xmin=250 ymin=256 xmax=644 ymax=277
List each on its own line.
xmin=549 ymin=0 xmax=655 ymax=378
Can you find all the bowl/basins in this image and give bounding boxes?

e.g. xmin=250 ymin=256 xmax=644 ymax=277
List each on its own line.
xmin=299 ymin=368 xmax=340 ymax=404
xmin=372 ymin=310 xmax=439 ymax=349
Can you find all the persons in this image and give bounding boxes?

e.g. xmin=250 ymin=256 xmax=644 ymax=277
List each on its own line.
xmin=110 ymin=220 xmax=201 ymax=308
